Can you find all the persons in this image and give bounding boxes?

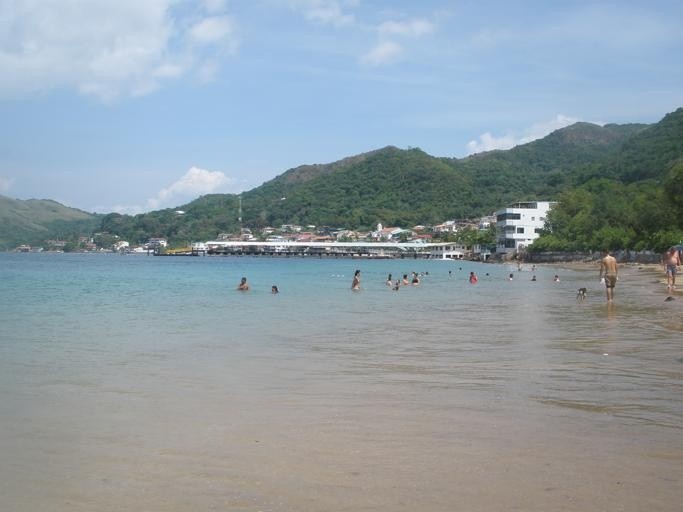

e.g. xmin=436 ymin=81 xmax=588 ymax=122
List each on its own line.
xmin=267 ymin=284 xmax=281 ymax=296
xmin=381 ymin=259 xmax=590 ymax=304
xmin=662 ymin=246 xmax=683 ymax=291
xmin=596 ymin=250 xmax=619 ymax=304
xmin=233 ymin=277 xmax=249 ymax=291
xmin=349 ymin=271 xmax=361 ymax=293
